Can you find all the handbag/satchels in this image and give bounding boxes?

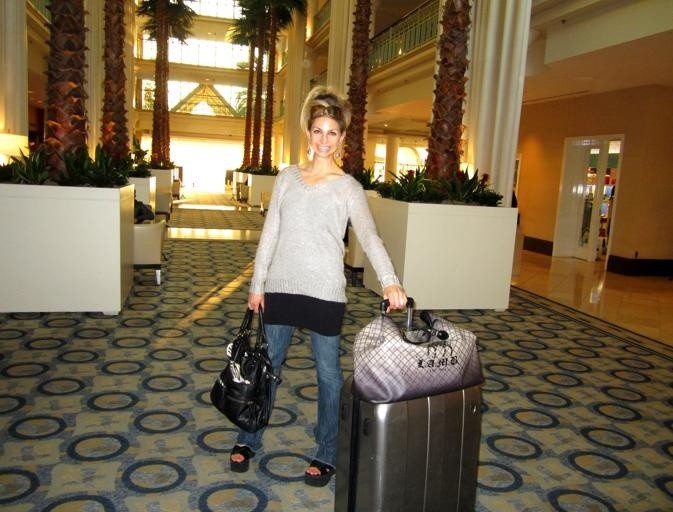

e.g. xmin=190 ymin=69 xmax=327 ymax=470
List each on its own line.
xmin=210 ymin=304 xmax=282 ymax=433
xmin=353 ymin=311 xmax=483 ymax=404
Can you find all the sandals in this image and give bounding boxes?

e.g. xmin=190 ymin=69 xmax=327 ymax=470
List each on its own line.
xmin=230 ymin=445 xmax=256 ymax=474
xmin=304 ymin=460 xmax=335 ymax=487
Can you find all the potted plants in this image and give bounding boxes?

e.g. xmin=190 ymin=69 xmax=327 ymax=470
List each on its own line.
xmin=0 ymin=0 xmax=183 ymax=317
xmin=227 ymin=1 xmax=520 ymax=319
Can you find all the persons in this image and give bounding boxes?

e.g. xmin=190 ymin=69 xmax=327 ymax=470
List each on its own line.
xmin=134 ymin=189 xmax=154 ymax=224
xmin=228 ymin=85 xmax=410 ymax=488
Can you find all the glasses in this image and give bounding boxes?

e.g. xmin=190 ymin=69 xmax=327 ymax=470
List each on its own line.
xmin=310 ymin=105 xmax=343 ymax=120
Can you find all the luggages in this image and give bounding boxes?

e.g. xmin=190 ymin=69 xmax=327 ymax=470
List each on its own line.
xmin=334 ymin=297 xmax=483 ymax=512
